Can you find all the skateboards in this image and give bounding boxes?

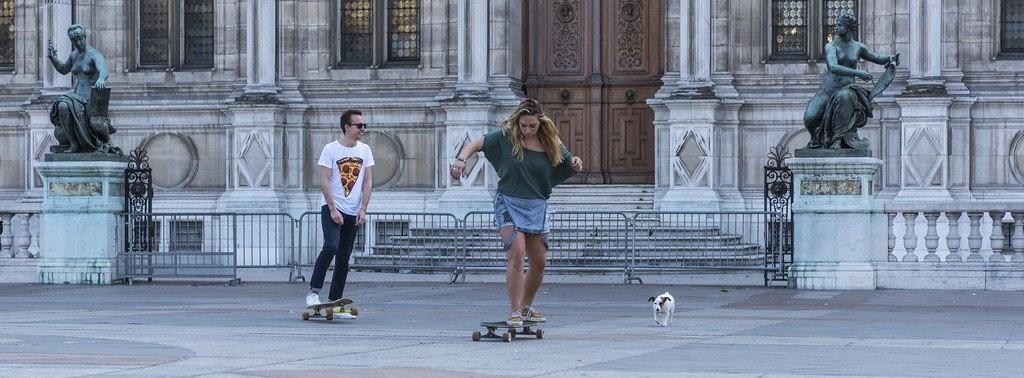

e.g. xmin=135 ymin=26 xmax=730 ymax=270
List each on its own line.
xmin=301 ymin=298 xmax=358 ymax=321
xmin=471 ymin=321 xmax=547 ymax=342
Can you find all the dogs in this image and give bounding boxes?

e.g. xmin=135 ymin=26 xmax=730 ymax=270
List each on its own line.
xmin=648 ymin=292 xmax=674 ymax=327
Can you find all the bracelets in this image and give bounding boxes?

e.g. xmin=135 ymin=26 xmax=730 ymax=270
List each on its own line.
xmin=457 ymin=157 xmax=466 ymax=164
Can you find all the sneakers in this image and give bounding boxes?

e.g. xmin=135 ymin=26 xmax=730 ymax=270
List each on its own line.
xmin=507 ymin=310 xmax=523 ymax=326
xmin=332 ymin=306 xmax=355 ymax=320
xmin=305 ymin=292 xmax=321 ymax=305
xmin=521 ymin=306 xmax=546 ymax=322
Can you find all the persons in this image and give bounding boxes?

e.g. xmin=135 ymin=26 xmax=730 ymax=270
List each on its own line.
xmin=305 ymin=110 xmax=375 ymax=320
xmin=451 ymin=98 xmax=582 ymax=326
xmin=47 ymin=24 xmax=116 ymax=153
xmin=803 ymin=12 xmax=901 ymax=149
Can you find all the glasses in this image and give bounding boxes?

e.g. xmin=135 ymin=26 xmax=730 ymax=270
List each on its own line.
xmin=346 ymin=123 xmax=367 ymax=129
